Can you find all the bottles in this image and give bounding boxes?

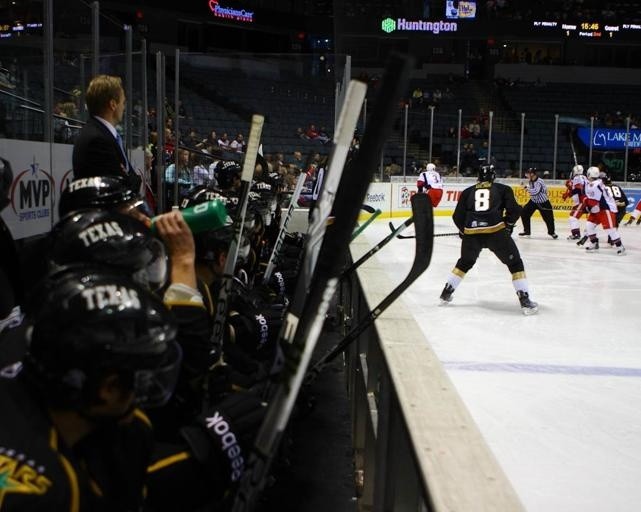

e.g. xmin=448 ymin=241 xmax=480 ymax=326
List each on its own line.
xmin=151 ymin=201 xmax=227 ymax=237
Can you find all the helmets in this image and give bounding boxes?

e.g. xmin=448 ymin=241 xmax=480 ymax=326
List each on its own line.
xmin=180 ymin=184 xmax=234 ymax=247
xmin=47 ymin=207 xmax=168 ymax=293
xmin=252 ymin=181 xmax=277 ymax=210
xmin=570 ymin=164 xmax=615 ymax=184
xmin=426 ymin=163 xmax=436 ymax=172
xmin=248 ymin=191 xmax=272 ymax=225
xmin=213 ymin=159 xmax=242 ymax=188
xmin=525 ymin=167 xmax=536 ymax=173
xmin=230 ymin=208 xmax=265 ymax=247
xmin=543 ymin=171 xmax=549 ymax=176
xmin=57 ymin=175 xmax=145 ymax=219
xmin=477 ymin=163 xmax=496 ymax=183
xmin=202 ymin=228 xmax=256 ymax=284
xmin=452 ymin=166 xmax=457 ymax=170
xmin=28 ymin=264 xmax=182 ymax=412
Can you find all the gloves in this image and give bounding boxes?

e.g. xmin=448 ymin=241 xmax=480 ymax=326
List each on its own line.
xmin=268 ymin=270 xmax=299 ymax=296
xmin=504 ymin=223 xmax=514 ymax=236
xmin=458 ymin=228 xmax=464 ymax=239
xmin=169 ymin=387 xmax=269 ymax=497
xmin=565 ymin=180 xmax=572 ymax=188
xmin=234 ymin=305 xmax=283 ymax=354
xmin=562 ymin=192 xmax=568 ymax=201
xmin=579 ymin=194 xmax=588 ymax=204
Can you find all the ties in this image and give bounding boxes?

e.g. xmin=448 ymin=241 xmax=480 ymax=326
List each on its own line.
xmin=115 ymin=130 xmax=129 ymax=174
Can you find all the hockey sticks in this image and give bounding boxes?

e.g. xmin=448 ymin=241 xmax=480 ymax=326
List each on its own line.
xmin=361 ymin=204 xmax=377 ymax=213
xmin=389 ymin=221 xmax=462 ymax=238
xmin=242 ymin=145 xmax=269 ymax=189
xmin=306 ymin=193 xmax=434 ymax=387
xmin=569 ymin=125 xmax=578 ymax=166
xmin=538 ymin=204 xmax=588 ymax=212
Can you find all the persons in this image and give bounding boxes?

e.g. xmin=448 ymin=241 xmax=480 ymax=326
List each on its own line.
xmin=2 ymin=56 xmax=330 ymax=511
xmin=483 ymin=46 xmax=552 ymax=64
xmin=415 ymin=160 xmax=443 ymax=207
xmin=348 ymin=75 xmax=504 ymax=181
xmin=518 ymin=165 xmax=641 ymax=254
xmin=480 ymin=76 xmax=640 ymax=182
xmin=437 ymin=164 xmax=539 ymax=317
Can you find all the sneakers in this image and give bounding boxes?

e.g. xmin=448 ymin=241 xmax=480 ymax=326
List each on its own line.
xmin=635 ymin=219 xmax=640 ymax=225
xmin=440 ymin=283 xmax=454 ymax=302
xmin=623 ymin=220 xmax=633 ymax=226
xmin=519 ymin=229 xmax=626 ymax=255
xmin=515 ymin=289 xmax=538 ymax=316
